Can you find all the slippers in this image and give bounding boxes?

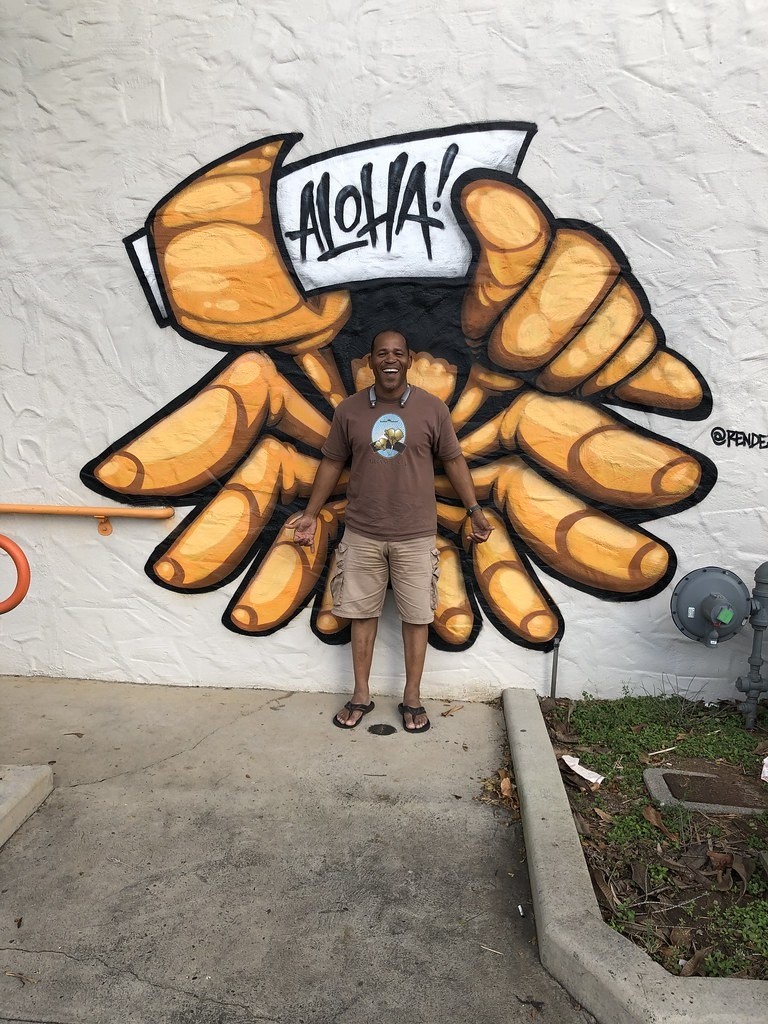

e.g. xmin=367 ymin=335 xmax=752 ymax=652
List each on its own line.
xmin=398 ymin=703 xmax=430 ymax=733
xmin=333 ymin=701 xmax=375 ymax=729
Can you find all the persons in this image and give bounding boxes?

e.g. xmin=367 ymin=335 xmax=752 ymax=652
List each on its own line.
xmin=284 ymin=328 xmax=495 ymax=734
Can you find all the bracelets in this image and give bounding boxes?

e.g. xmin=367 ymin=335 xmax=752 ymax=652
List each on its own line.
xmin=466 ymin=504 xmax=482 ymax=517
